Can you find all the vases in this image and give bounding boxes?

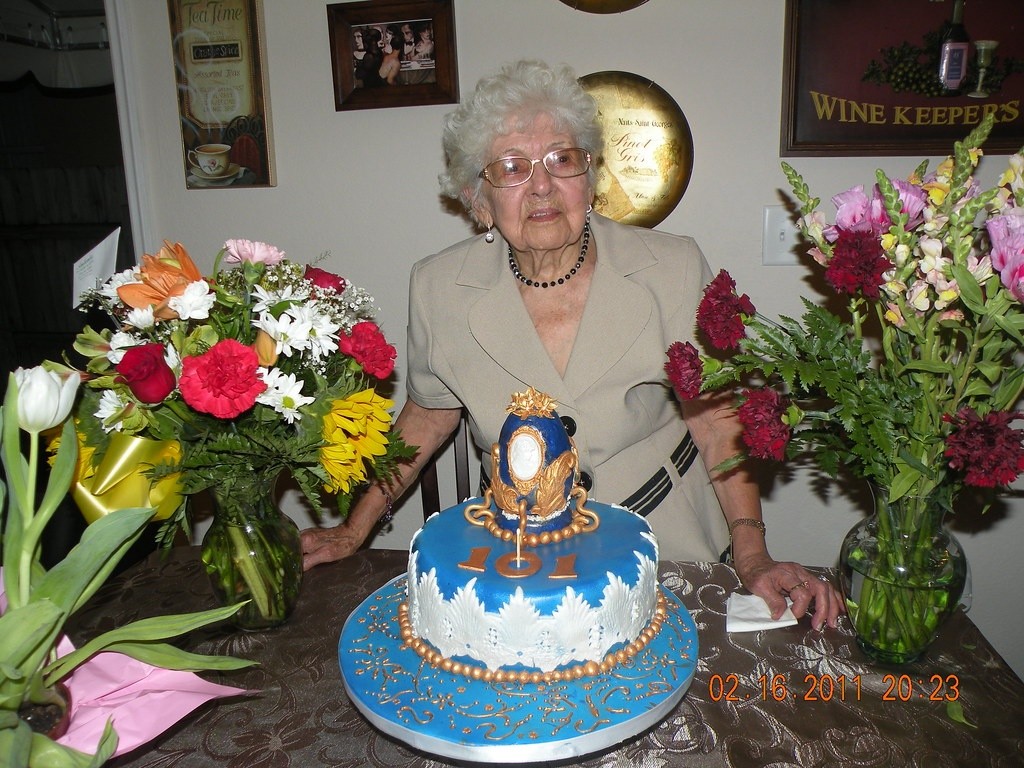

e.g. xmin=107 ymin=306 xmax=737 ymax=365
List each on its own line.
xmin=838 ymin=476 xmax=968 ymax=665
xmin=203 ymin=464 xmax=305 ymax=633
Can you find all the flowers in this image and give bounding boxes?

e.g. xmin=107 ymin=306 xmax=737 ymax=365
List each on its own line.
xmin=0 ymin=236 xmax=421 ymax=768
xmin=661 ymin=112 xmax=1022 ymax=650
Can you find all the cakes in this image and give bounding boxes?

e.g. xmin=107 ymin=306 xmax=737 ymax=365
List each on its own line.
xmin=398 ymin=386 xmax=666 ymax=684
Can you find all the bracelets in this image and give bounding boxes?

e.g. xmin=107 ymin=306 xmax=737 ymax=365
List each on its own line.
xmin=380 ymin=492 xmax=393 ymax=523
xmin=729 ymin=518 xmax=766 ymax=537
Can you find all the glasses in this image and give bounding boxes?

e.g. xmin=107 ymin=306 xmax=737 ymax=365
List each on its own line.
xmin=479 ymin=147 xmax=592 ymax=188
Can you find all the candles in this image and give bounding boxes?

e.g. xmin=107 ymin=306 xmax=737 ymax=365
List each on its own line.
xmin=517 ymin=528 xmax=521 ymax=567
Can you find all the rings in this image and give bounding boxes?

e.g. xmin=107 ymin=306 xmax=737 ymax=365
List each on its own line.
xmin=790 ymin=581 xmax=810 ymax=590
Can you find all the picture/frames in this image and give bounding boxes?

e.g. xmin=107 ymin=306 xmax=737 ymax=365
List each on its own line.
xmin=326 ymin=0 xmax=460 ymax=112
xmin=780 ymin=0 xmax=1024 ymax=160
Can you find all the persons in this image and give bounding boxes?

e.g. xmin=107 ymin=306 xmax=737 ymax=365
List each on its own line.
xmin=353 ymin=24 xmax=433 ymax=86
xmin=298 ymin=59 xmax=846 ymax=632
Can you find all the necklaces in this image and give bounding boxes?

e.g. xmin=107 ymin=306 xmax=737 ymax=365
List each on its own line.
xmin=508 ymin=222 xmax=590 ymax=289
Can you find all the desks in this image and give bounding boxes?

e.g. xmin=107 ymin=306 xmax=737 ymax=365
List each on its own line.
xmin=54 ymin=548 xmax=1024 ymax=768
